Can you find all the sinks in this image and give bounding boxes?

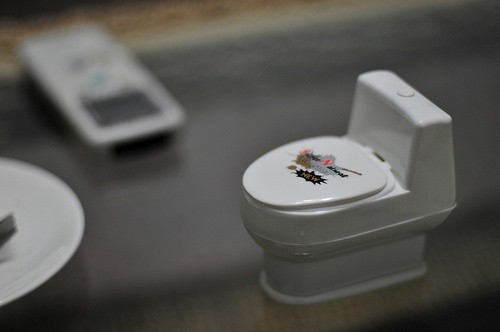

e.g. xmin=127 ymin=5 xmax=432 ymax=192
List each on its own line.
xmin=0 ymin=153 xmax=87 ymax=307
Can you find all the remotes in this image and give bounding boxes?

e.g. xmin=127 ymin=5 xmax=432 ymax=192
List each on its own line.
xmin=14 ymin=17 xmax=187 ymax=148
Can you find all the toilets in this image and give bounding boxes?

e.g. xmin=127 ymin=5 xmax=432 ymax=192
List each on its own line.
xmin=239 ymin=69 xmax=457 ymax=306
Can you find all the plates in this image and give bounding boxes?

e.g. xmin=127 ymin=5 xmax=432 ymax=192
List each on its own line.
xmin=0 ymin=155 xmax=85 ymax=307
